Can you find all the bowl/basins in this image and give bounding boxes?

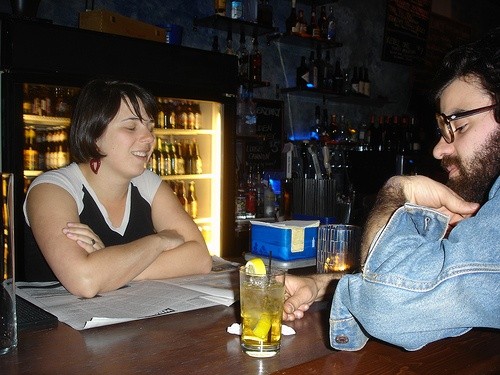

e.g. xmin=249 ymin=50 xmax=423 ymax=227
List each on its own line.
xmin=155 ymin=24 xmax=184 ymax=45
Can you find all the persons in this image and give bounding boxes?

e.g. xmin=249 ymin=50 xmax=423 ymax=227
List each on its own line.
xmin=271 ymin=49 xmax=500 ymax=353
xmin=7 ymin=79 xmax=213 ymax=298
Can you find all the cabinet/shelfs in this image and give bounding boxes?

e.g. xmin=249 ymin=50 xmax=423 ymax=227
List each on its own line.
xmin=201 ymin=16 xmax=371 ymax=105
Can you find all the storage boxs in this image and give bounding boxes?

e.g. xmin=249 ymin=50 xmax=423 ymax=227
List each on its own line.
xmin=79 ymin=10 xmax=167 ymax=42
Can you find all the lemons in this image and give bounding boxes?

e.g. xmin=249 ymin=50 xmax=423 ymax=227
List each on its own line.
xmin=253 ymin=315 xmax=271 ymax=338
xmin=245 ymin=257 xmax=266 ymax=276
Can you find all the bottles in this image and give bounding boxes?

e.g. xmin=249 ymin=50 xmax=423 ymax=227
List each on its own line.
xmin=211 ymin=28 xmax=262 ymax=84
xmin=231 ymin=0 xmax=245 ymax=20
xmin=235 ymin=162 xmax=275 ymax=220
xmin=20 ymin=96 xmax=202 ymax=220
xmin=214 ymin=0 xmax=227 ymax=16
xmin=311 ymin=105 xmax=422 ymax=152
xmin=296 ymin=48 xmax=371 ymax=98
xmin=285 ymin=0 xmax=337 ymax=42
xmin=257 ymin=0 xmax=273 ymax=26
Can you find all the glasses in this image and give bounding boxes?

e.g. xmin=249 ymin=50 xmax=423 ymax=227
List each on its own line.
xmin=434 ymin=102 xmax=500 ymax=144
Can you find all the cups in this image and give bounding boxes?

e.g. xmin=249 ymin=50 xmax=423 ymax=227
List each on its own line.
xmin=239 ymin=265 xmax=289 ymax=359
xmin=317 ymin=224 xmax=361 ymax=275
